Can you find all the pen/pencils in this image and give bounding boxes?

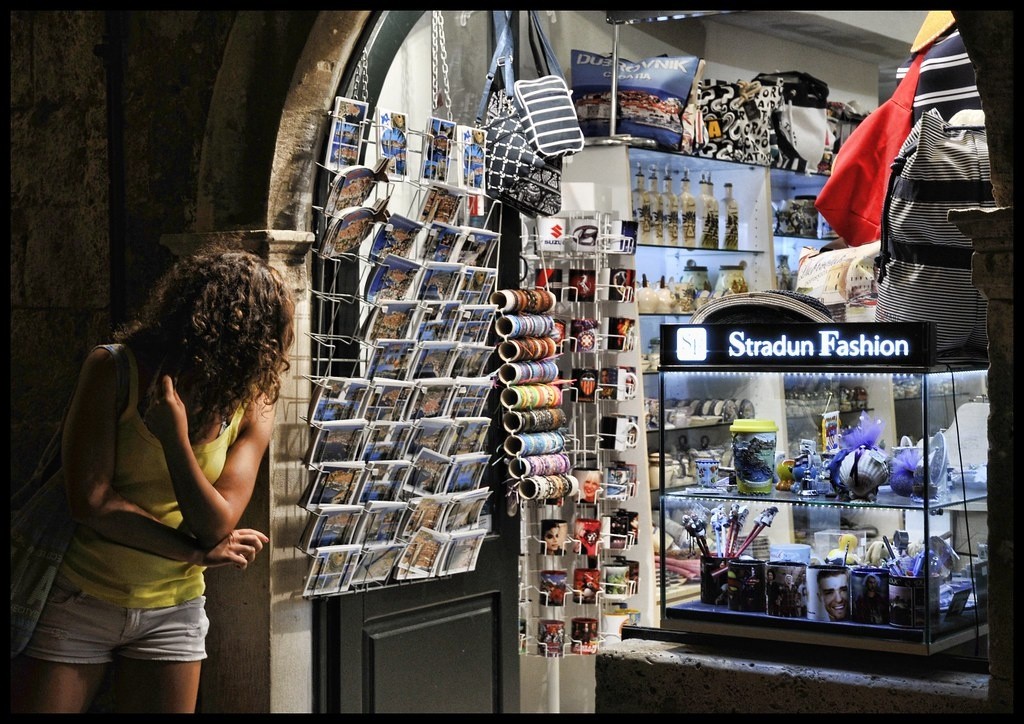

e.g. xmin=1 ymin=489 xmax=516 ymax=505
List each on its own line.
xmin=881 ymin=535 xmax=940 ymax=578
xmin=842 ymin=540 xmax=850 ymax=566
xmin=681 ymin=505 xmax=780 ymax=559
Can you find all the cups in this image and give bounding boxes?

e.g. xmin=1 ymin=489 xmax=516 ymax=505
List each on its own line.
xmin=851 ymin=568 xmax=889 ymax=624
xmin=727 ymin=559 xmax=767 ymax=612
xmin=520 ymin=214 xmax=641 ymax=658
xmin=764 ymin=561 xmax=808 ymax=617
xmin=889 ymin=572 xmax=939 ymax=629
xmin=730 ymin=418 xmax=779 ymax=494
xmin=806 ymin=564 xmax=851 ymax=621
xmin=700 ymin=553 xmax=741 ymax=606
xmin=696 ymin=460 xmax=719 ymax=487
xmin=769 ymin=544 xmax=810 ymax=564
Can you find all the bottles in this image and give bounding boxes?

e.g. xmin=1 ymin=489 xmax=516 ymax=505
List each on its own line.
xmin=631 ymin=165 xmax=739 ymax=250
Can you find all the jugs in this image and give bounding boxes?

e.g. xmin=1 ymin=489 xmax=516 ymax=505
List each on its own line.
xmin=890 ymin=447 xmax=922 ymax=496
xmin=773 ymin=199 xmax=800 ymax=236
xmin=637 ymin=266 xmax=747 ymax=314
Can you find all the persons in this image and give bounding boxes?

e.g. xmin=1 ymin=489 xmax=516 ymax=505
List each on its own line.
xmin=859 ymin=575 xmax=889 ymax=623
xmin=798 ymin=572 xmax=807 ymax=618
xmin=575 ymin=470 xmax=603 ymax=504
xmin=778 ymin=574 xmax=803 ymax=618
xmin=541 ymin=522 xmax=567 ymax=555
xmin=344 ymin=103 xmax=363 ymax=125
xmin=816 ymin=570 xmax=853 ymax=622
xmin=765 ymin=572 xmax=781 ymax=616
xmin=18 ymin=246 xmax=294 ymax=717
xmin=628 ymin=513 xmax=639 ymax=545
xmin=741 ymin=566 xmax=759 ymax=608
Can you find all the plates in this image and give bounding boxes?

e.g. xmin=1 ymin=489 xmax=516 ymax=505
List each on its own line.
xmin=929 ymin=432 xmax=946 ymax=486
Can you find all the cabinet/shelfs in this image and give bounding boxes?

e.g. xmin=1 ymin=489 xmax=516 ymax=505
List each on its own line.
xmin=556 ymin=146 xmax=992 ymax=657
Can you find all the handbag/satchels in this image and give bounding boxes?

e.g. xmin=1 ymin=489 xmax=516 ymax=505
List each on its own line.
xmin=873 ymin=108 xmax=997 ymax=359
xmin=11 ymin=343 xmax=130 ymax=662
xmin=571 ymin=44 xmax=830 ymax=173
xmin=474 ymin=12 xmax=584 ymax=220
xmin=814 ymin=37 xmax=937 ymax=246
